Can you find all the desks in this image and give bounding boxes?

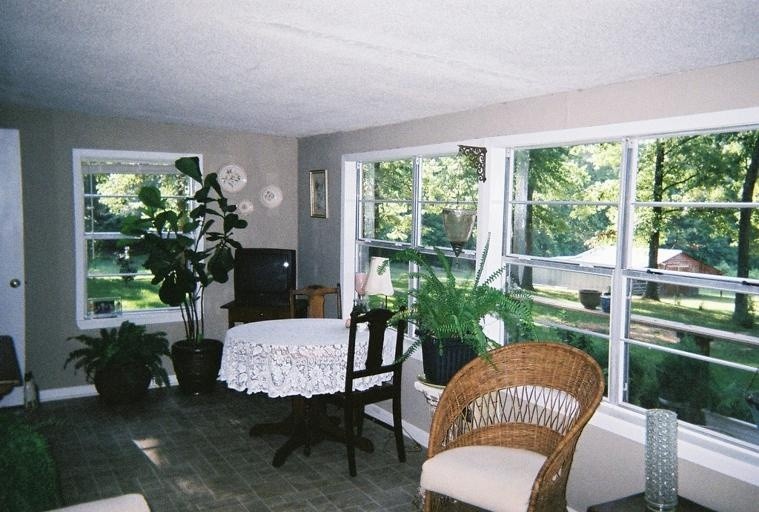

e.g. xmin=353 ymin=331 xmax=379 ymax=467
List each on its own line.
xmin=221 ymin=301 xmax=307 ymax=392
xmin=216 ymin=319 xmax=398 ymax=468
xmin=587 ymin=492 xmax=718 ymax=512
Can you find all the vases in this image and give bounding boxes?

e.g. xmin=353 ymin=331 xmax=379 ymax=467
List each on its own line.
xmin=579 ymin=289 xmax=602 ymax=309
xmin=600 ymin=296 xmax=610 ymax=313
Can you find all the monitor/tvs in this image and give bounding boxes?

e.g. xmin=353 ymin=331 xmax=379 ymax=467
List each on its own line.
xmin=234 ymin=247 xmax=296 ymax=307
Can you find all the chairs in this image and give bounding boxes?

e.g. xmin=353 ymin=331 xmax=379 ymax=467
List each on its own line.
xmin=420 ymin=343 xmax=605 ymax=512
xmin=303 ymin=305 xmax=407 ymax=476
xmin=288 ymin=283 xmax=342 ymax=318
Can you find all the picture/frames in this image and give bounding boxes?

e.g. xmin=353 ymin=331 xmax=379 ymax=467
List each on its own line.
xmin=310 ymin=169 xmax=329 ymax=219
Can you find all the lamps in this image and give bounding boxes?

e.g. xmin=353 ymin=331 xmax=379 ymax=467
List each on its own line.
xmin=443 ymin=145 xmax=488 ymax=257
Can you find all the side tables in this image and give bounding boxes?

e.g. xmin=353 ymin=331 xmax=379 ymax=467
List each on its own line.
xmin=413 ymin=381 xmax=479 ymax=512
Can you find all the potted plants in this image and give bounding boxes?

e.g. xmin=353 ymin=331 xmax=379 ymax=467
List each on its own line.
xmin=63 ymin=319 xmax=172 ymax=404
xmin=376 ymin=233 xmax=540 ymax=386
xmin=116 ymin=157 xmax=247 ymax=396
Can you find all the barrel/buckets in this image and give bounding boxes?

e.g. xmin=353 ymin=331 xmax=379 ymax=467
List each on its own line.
xmin=442 ymin=208 xmax=476 ymax=242
